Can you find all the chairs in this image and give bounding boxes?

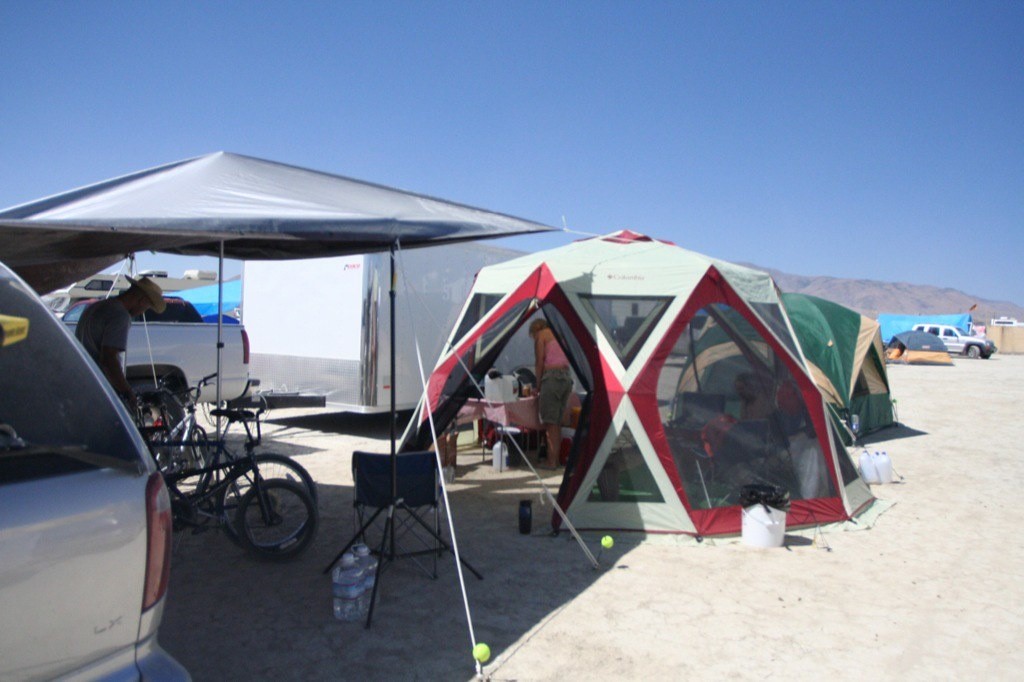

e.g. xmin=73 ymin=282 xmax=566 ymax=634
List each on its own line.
xmin=350 ymin=449 xmax=450 ymax=582
xmin=685 ymin=413 xmax=737 ymax=509
xmin=661 ymin=390 xmax=725 ymax=434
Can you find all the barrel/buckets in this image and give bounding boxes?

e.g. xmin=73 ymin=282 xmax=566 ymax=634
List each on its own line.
xmin=561 ymin=427 xmax=576 ymax=465
xmin=485 ymin=373 xmax=519 ymax=402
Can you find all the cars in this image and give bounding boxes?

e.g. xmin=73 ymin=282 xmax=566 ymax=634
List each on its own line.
xmin=1 ymin=260 xmax=194 ymax=682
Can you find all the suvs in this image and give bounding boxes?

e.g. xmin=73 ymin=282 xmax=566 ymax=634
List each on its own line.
xmin=912 ymin=323 xmax=995 ymax=359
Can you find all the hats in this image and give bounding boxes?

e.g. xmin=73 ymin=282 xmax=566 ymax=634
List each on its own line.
xmin=124 ymin=274 xmax=166 ymax=314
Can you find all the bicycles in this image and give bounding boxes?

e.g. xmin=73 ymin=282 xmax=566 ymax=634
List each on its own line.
xmin=132 ymin=370 xmax=320 ymax=565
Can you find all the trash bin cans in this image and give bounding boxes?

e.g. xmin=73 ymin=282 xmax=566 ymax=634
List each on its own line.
xmin=496 ymin=428 xmax=523 ymax=468
xmin=739 ymin=485 xmax=792 ymax=548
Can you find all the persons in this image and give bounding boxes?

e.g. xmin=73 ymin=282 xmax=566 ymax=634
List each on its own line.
xmin=529 ymin=318 xmax=575 ymax=470
xmin=75 ymin=275 xmax=168 ymax=406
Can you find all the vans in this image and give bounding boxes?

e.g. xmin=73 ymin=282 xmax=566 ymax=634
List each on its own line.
xmin=39 ymin=269 xmax=237 ymax=323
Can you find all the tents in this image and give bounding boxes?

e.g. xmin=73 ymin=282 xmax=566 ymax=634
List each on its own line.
xmin=392 ymin=230 xmax=973 ymax=543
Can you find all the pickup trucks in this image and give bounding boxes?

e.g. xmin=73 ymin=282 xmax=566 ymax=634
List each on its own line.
xmin=65 ymin=321 xmax=251 ymax=450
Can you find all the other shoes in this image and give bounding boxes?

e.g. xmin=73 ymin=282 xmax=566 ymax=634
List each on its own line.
xmin=534 ymin=457 xmax=562 ymax=471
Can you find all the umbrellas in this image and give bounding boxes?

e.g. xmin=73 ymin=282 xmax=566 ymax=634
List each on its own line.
xmin=0 ymin=150 xmax=560 ymax=477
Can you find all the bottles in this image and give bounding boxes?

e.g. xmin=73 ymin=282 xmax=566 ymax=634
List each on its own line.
xmin=492 ymin=438 xmax=508 ymax=471
xmin=332 ymin=553 xmax=364 ymax=621
xmin=350 ymin=543 xmax=377 ymax=612
xmin=859 ymin=449 xmax=893 ymax=483
xmin=442 ymin=465 xmax=454 ymax=484
xmin=519 ymin=500 xmax=532 ymax=535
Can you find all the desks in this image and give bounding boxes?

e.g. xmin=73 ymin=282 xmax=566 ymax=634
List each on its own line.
xmin=443 ymin=394 xmax=582 ymax=468
